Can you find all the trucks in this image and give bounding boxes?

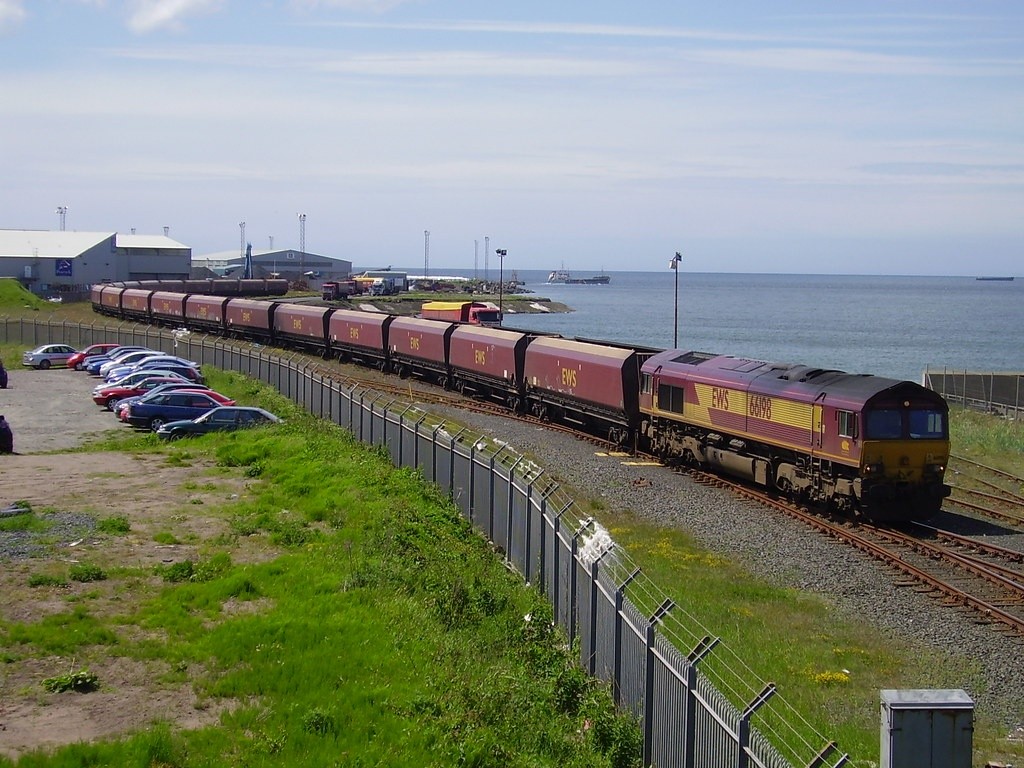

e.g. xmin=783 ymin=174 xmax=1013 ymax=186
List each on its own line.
xmin=321 ymin=276 xmax=405 ymax=300
xmin=420 ymin=300 xmax=504 ymax=329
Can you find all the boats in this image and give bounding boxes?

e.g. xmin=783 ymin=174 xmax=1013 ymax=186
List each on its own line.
xmin=564 ymin=265 xmax=611 ymax=284
xmin=548 ymin=272 xmax=569 ymax=282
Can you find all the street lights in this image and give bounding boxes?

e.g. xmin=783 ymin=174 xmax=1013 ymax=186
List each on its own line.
xmin=668 ymin=251 xmax=682 ymax=349
xmin=495 ymin=249 xmax=507 ymax=327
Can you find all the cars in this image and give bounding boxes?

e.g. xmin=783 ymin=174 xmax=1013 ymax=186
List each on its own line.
xmin=119 ymin=388 xmax=236 ymax=423
xmin=126 ymin=391 xmax=225 ymax=433
xmin=113 ymin=384 xmax=212 ymax=419
xmin=92 ymin=371 xmax=188 ymax=397
xmin=92 ymin=379 xmax=185 ymax=412
xmin=82 ymin=346 xmax=206 ymax=386
xmin=156 ymin=406 xmax=284 ymax=442
xmin=66 ymin=343 xmax=121 ymax=372
xmin=23 ymin=344 xmax=82 ymax=371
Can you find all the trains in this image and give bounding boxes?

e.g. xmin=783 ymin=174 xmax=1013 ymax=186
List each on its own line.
xmin=89 ymin=277 xmax=952 ymax=526
